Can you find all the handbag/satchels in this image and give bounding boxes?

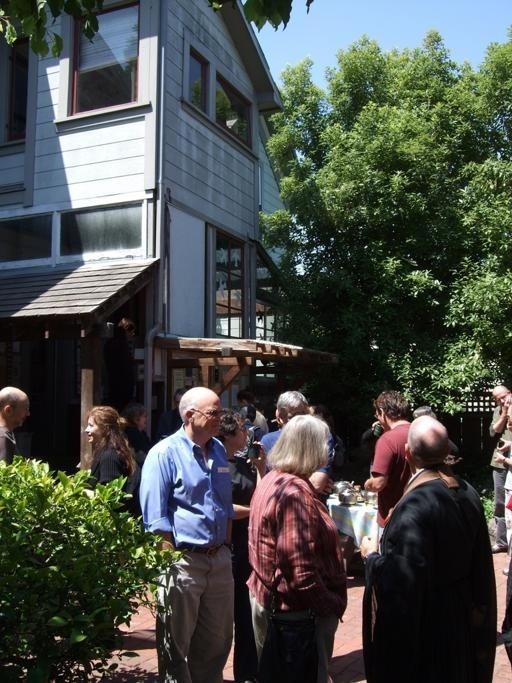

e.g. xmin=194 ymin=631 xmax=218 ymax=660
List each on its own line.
xmin=258 ymin=612 xmax=319 ymax=683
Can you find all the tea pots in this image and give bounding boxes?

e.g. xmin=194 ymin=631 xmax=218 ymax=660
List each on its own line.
xmin=338 ymin=480 xmax=358 ymax=504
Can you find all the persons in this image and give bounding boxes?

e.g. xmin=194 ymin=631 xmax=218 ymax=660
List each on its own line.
xmin=363 ymin=390 xmax=459 ymax=531
xmin=214 ymin=408 xmax=266 ymax=683
xmin=237 ymin=388 xmax=346 ymax=496
xmin=159 ymin=389 xmax=189 ymax=441
xmin=122 ymin=400 xmax=150 ymax=454
xmin=140 ymin=387 xmax=235 ymax=683
xmin=360 ymin=415 xmax=498 ymax=683
xmin=489 ymin=385 xmax=512 ymax=575
xmin=86 ymin=405 xmax=135 ymax=492
xmin=246 ymin=415 xmax=348 ymax=683
xmin=0 ymin=386 xmax=30 ymax=466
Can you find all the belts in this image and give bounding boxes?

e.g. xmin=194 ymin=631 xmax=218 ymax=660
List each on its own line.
xmin=178 ymin=543 xmax=225 ymax=555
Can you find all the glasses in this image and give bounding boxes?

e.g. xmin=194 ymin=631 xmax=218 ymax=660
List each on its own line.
xmin=191 ymin=408 xmax=226 ymax=415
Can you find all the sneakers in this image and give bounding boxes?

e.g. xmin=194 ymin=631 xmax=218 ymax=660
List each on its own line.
xmin=492 ymin=544 xmax=506 ymax=554
